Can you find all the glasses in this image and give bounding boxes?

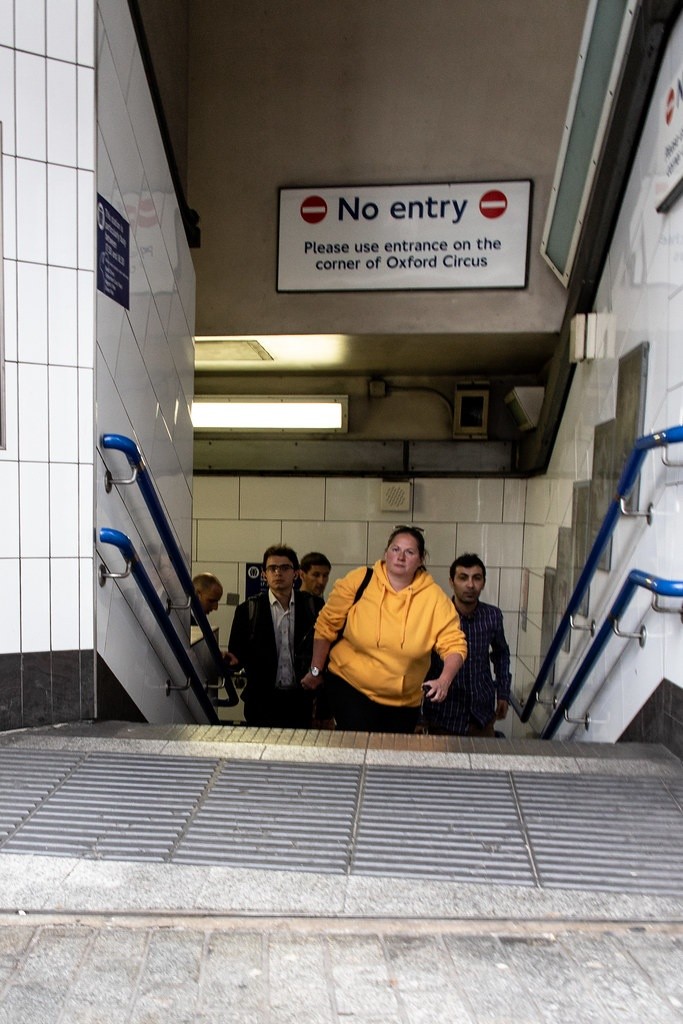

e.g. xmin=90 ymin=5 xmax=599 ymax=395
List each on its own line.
xmin=392 ymin=525 xmax=424 ymax=538
xmin=265 ymin=564 xmax=294 ymax=572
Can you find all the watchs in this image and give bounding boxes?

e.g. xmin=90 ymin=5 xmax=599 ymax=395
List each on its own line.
xmin=310 ymin=666 xmax=321 ymax=677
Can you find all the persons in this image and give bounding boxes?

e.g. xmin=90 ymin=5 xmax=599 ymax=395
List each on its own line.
xmin=191 ymin=573 xmax=223 ymax=616
xmin=298 ymin=551 xmax=331 ymax=611
xmin=223 ymin=543 xmax=327 ymax=729
xmin=425 ymin=552 xmax=512 ymax=737
xmin=299 ymin=524 xmax=468 ymax=733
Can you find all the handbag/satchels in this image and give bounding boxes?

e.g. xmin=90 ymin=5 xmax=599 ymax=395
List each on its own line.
xmin=312 ymin=670 xmax=334 ymax=721
